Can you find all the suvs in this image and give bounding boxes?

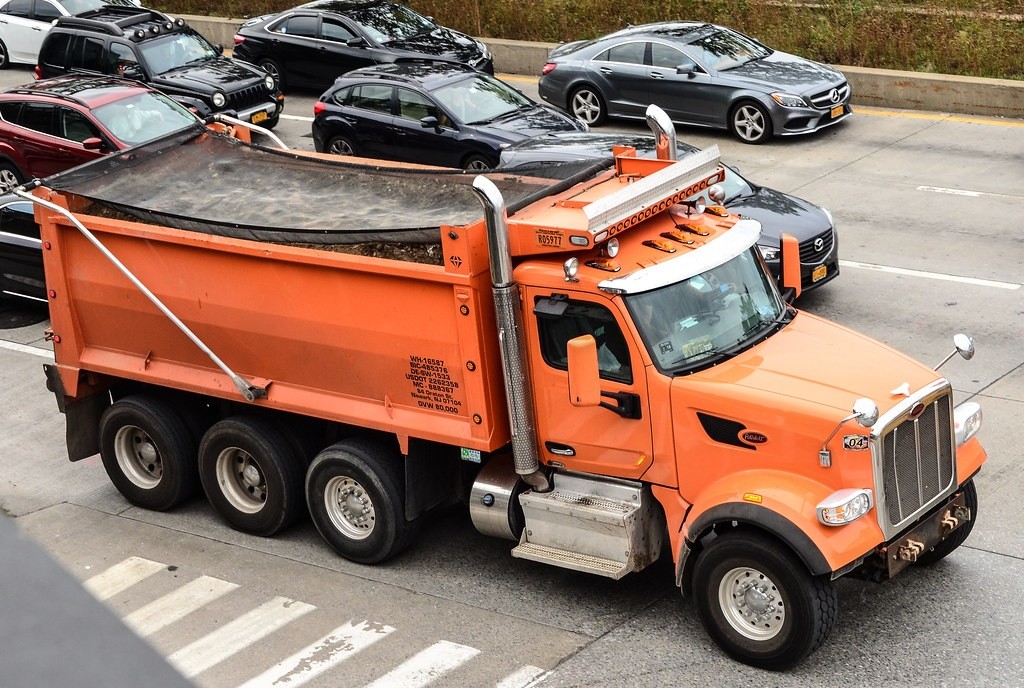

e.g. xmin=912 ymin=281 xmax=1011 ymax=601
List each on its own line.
xmin=312 ymin=58 xmax=591 ymax=171
xmin=35 ymin=7 xmax=284 ymax=134
xmin=0 ymin=71 xmax=205 ymax=219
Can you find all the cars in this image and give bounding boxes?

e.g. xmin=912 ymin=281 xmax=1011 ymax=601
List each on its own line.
xmin=0 ymin=196 xmax=49 ymax=312
xmin=0 ymin=0 xmax=142 ymax=69
xmin=538 ymin=21 xmax=853 ymax=145
xmin=495 ymin=133 xmax=840 ymax=293
xmin=232 ymin=0 xmax=495 ymax=94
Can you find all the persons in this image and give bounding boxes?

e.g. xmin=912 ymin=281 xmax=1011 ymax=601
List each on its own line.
xmin=441 ymin=87 xmax=485 ymax=132
xmin=637 ymin=282 xmax=738 ymax=340
xmin=108 ymin=106 xmax=163 ymax=142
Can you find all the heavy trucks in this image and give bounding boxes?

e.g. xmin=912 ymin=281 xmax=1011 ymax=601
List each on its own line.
xmin=12 ymin=112 xmax=987 ymax=672
